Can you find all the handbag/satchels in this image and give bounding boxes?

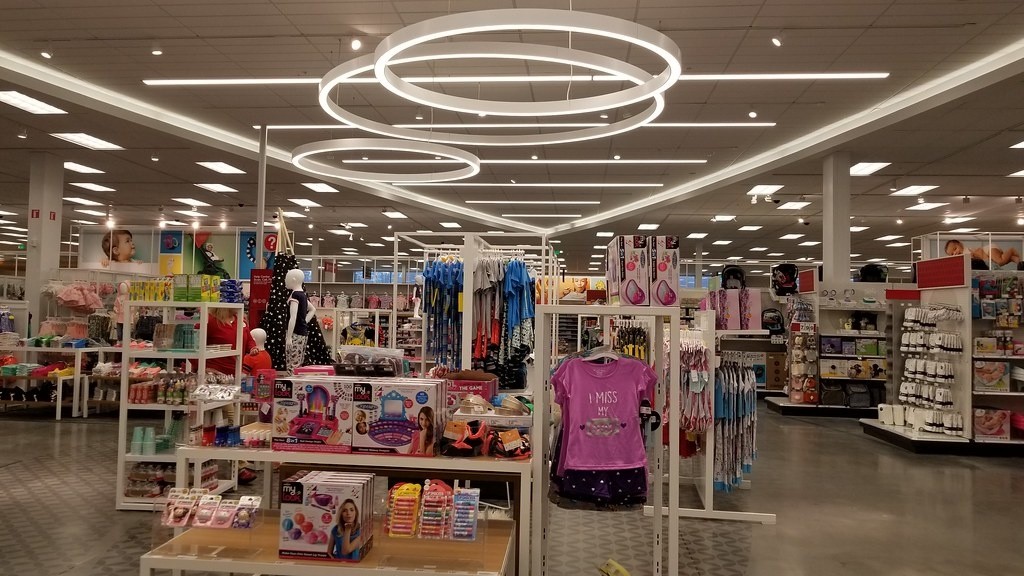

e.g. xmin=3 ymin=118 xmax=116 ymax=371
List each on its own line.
xmin=821 ymin=381 xmax=886 ymax=408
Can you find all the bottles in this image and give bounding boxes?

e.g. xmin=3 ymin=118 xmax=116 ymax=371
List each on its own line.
xmin=240 ymin=430 xmax=271 ymax=448
xmin=187 ymin=423 xmax=241 ymax=448
xmin=157 ymin=378 xmax=184 ymax=405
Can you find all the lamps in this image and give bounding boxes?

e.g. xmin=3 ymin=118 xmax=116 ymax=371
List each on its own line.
xmin=962 ymin=196 xmax=969 ymax=203
xmin=292 ymin=131 xmax=482 ymax=182
xmin=1014 ymin=197 xmax=1022 ymax=204
xmin=318 ymin=43 xmax=666 ymax=145
xmin=374 ymin=0 xmax=682 ymax=118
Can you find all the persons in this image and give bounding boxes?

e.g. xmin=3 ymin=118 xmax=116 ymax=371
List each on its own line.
xmin=101 ymin=229 xmax=142 ymax=265
xmin=945 ymin=240 xmax=1021 ymax=266
xmin=205 ymin=243 xmax=219 ymax=260
xmin=327 ymin=499 xmax=361 ymax=561
xmin=283 ymin=269 xmax=317 ymax=376
xmin=563 ymin=278 xmax=588 ymax=300
xmin=356 ymin=411 xmax=367 ymax=434
xmin=982 ymin=412 xmax=1007 ymax=431
xmin=243 ymin=328 xmax=272 ymax=395
xmin=983 ymin=363 xmax=1006 ymax=382
xmin=190 ymin=267 xmax=258 ymax=485
xmin=114 ymin=280 xmax=130 ymax=342
xmin=408 ymin=406 xmax=433 ymax=457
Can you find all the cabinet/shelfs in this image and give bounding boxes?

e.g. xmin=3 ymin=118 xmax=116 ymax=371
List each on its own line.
xmin=972 ymin=354 xmax=1024 ymax=444
xmin=115 ymin=300 xmax=244 ymax=511
xmin=819 ymin=308 xmax=887 ymax=408
xmin=295 ymin=254 xmax=437 ymax=363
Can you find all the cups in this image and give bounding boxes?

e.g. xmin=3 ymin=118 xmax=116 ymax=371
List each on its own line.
xmin=212 ymin=409 xmax=224 ymax=426
xmin=130 ymin=426 xmax=156 ymax=456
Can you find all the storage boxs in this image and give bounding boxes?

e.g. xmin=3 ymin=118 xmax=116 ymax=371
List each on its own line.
xmin=847 ymin=360 xmax=869 ymax=378
xmin=648 ymin=234 xmax=680 ymax=308
xmin=973 ymin=360 xmax=1010 ymax=393
xmin=867 ymin=359 xmax=886 ymax=378
xmin=274 ymin=370 xmax=500 ymax=458
xmin=706 ymin=288 xmax=761 ymax=330
xmin=972 ymin=405 xmax=1010 ymax=442
xmin=767 ymin=352 xmax=787 ymax=389
xmin=819 ymin=358 xmax=847 ymax=377
xmin=972 ymin=338 xmax=997 ymax=354
xmin=278 ymin=470 xmax=374 ymax=563
xmin=745 ymin=352 xmax=766 ymax=387
xmin=606 ymin=235 xmax=649 ymax=306
xmin=129 ymin=275 xmax=220 ymax=303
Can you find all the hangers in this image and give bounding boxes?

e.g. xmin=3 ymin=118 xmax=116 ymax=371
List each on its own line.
xmin=663 ymin=328 xmax=706 ymax=353
xmin=309 ymin=291 xmax=403 ymax=299
xmin=0 ymin=306 xmax=12 ymax=316
xmin=425 ymin=250 xmax=462 ymax=265
xmin=582 ymin=341 xmax=623 ymax=365
xmin=717 ymin=352 xmax=756 ymax=376
xmin=40 ymin=280 xmax=161 ymax=330
xmin=479 ymin=249 xmax=524 ymax=263
xmin=617 ymin=320 xmax=650 ymax=339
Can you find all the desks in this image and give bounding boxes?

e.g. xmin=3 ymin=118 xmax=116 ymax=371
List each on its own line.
xmin=174 ymin=445 xmax=531 ymax=576
xmin=140 ymin=518 xmax=515 ymax=576
xmin=0 ymin=348 xmax=98 ymax=418
xmin=0 ymin=374 xmax=86 ymax=420
